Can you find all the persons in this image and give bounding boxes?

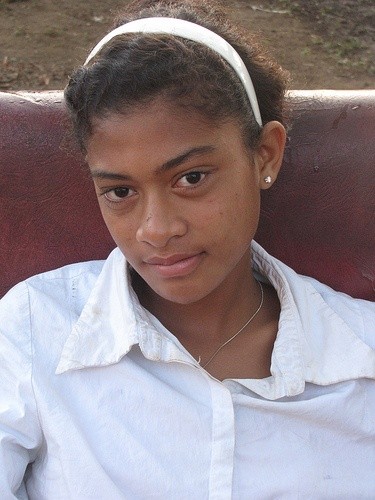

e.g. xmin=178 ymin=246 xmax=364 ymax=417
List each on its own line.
xmin=0 ymin=0 xmax=373 ymax=498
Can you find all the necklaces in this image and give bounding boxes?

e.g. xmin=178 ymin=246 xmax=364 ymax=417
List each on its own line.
xmin=197 ymin=281 xmax=263 ymax=370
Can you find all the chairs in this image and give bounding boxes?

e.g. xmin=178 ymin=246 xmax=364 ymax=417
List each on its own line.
xmin=1 ymin=90 xmax=375 ymax=302
